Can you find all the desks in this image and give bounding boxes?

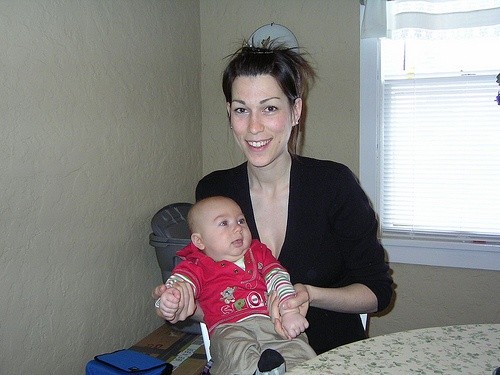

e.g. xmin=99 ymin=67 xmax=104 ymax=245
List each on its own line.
xmin=285 ymin=323 xmax=500 ymax=375
xmin=130 ymin=323 xmax=212 ymax=375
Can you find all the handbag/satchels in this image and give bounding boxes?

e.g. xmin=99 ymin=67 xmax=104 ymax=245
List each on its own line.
xmin=85 ymin=348 xmax=173 ymax=375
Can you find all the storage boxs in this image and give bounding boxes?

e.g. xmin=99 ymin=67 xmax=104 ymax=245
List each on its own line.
xmin=149 ymin=202 xmax=202 ymax=335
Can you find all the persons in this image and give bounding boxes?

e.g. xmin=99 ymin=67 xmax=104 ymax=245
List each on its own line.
xmin=151 ymin=36 xmax=395 ymax=375
xmin=159 ymin=196 xmax=317 ymax=375
